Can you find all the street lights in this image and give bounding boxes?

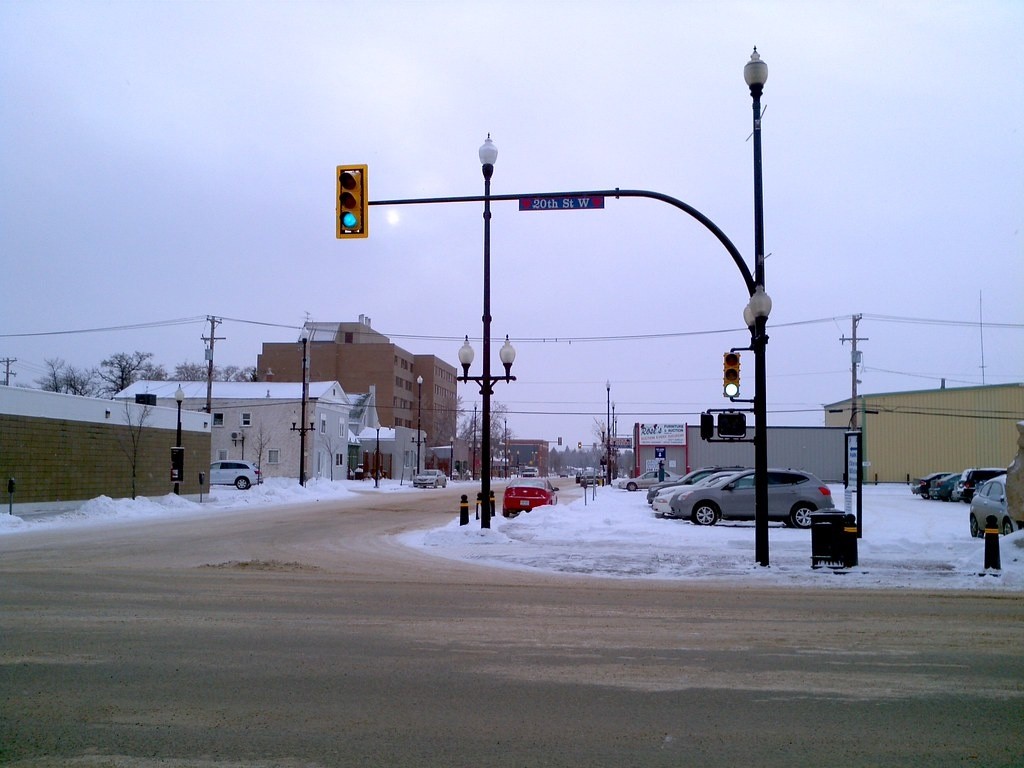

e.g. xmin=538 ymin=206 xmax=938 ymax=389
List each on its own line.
xmin=516 ymin=450 xmax=519 ymax=477
xmin=290 ymin=326 xmax=317 ymax=486
xmin=171 ymin=384 xmax=185 ymax=495
xmin=469 ymin=402 xmax=479 ymax=481
xmin=602 ymin=378 xmax=618 ymax=485
xmin=450 ymin=436 xmax=455 ymax=481
xmin=374 ymin=420 xmax=381 ymax=488
xmin=410 ymin=375 xmax=427 ymax=475
xmin=457 ymin=133 xmax=517 ymax=529
xmin=499 ymin=416 xmax=511 ymax=479
xmin=743 ymin=45 xmax=773 ymax=564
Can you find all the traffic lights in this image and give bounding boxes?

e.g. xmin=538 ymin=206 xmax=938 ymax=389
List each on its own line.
xmin=557 ymin=437 xmax=562 ymax=445
xmin=722 ymin=352 xmax=741 ymax=398
xmin=578 ymin=441 xmax=582 ymax=449
xmin=336 ymin=164 xmax=368 ymax=239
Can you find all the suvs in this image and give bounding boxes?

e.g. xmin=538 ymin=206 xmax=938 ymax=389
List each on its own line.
xmin=209 ymin=460 xmax=263 ymax=490
xmin=647 ymin=465 xmax=835 ymax=529
xmin=954 ymin=468 xmax=1007 ymax=504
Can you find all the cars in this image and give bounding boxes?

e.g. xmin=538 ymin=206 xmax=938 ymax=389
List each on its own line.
xmin=503 ymin=477 xmax=559 ymax=517
xmin=413 ymin=469 xmax=448 ymax=488
xmin=522 ymin=467 xmax=539 ymax=477
xmin=910 ymin=467 xmax=960 ymax=503
xmin=576 ymin=470 xmax=606 ymax=488
xmin=970 ymin=474 xmax=1024 ymax=538
xmin=618 ymin=469 xmax=684 ymax=491
xmin=560 ymin=472 xmax=568 ymax=478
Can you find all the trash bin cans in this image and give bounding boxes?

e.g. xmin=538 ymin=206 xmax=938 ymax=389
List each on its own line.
xmin=810 ymin=509 xmax=847 ymax=570
